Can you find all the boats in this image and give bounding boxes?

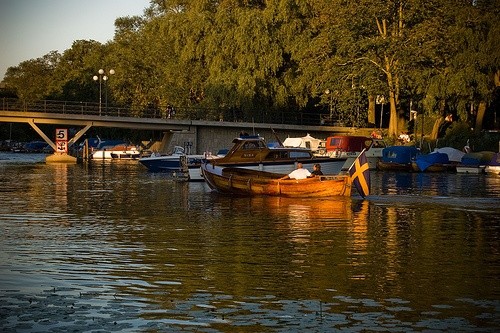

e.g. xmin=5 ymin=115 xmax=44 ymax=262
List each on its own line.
xmin=0 ymin=134 xmax=500 ymax=179
xmin=200 ymin=160 xmax=353 ymax=200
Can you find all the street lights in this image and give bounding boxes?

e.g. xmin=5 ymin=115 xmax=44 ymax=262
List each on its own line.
xmin=93 ymin=68 xmax=116 ymax=116
xmin=324 ymin=88 xmax=333 ymax=126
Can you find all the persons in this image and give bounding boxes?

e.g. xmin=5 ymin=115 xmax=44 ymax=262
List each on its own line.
xmin=280 ymin=162 xmax=314 ymax=180
xmin=311 ymin=165 xmax=324 ymax=181
